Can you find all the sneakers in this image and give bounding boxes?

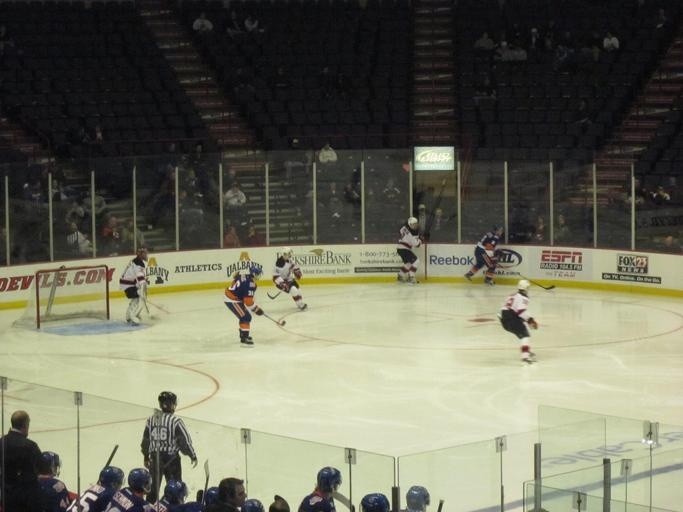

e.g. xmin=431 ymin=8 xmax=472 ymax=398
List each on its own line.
xmin=240 ymin=336 xmax=253 ymax=345
xmin=521 ymin=352 xmax=536 ymax=363
xmin=484 ymin=276 xmax=495 ymax=284
xmin=464 ymin=273 xmax=472 ymax=282
xmin=298 ymin=303 xmax=307 ymax=310
xmin=127 ymin=314 xmax=141 ymax=327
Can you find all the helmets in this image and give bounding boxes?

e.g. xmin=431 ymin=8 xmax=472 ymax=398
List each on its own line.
xmin=127 ymin=467 xmax=152 ymax=495
xmin=163 ymin=479 xmax=188 ymax=505
xmin=158 ymin=390 xmax=177 ymax=413
xmin=360 ymin=493 xmax=390 ymax=512
xmin=241 ymin=499 xmax=265 ymax=512
xmin=517 ymin=279 xmax=530 ymax=296
xmin=282 ymin=248 xmax=293 ymax=261
xmin=204 ymin=487 xmax=220 ymax=505
xmin=137 ymin=248 xmax=148 ymax=262
xmin=98 ymin=464 xmax=124 ymax=489
xmin=493 ymin=223 xmax=504 ymax=238
xmin=41 ymin=451 xmax=61 ymax=476
xmin=316 ymin=466 xmax=342 ymax=494
xmin=405 ymin=486 xmax=430 ymax=512
xmin=407 ymin=217 xmax=418 ymax=230
xmin=250 ymin=266 xmax=262 ymax=280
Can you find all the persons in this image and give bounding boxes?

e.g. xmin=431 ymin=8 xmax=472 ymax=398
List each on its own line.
xmin=280 ymin=140 xmax=390 ymax=241
xmin=272 ymin=248 xmax=308 ymax=311
xmin=395 ymin=216 xmax=424 ymax=284
xmin=193 ymin=11 xmax=214 ymax=34
xmin=531 ymin=178 xmax=676 ymax=248
xmin=193 ymin=468 xmax=438 ymax=512
xmin=497 ymin=279 xmax=538 ymax=362
xmin=140 ymin=391 xmax=198 ymax=502
xmin=417 ymin=203 xmax=434 ymax=241
xmin=68 ymin=466 xmax=124 ymax=512
xmin=120 ymin=247 xmax=150 ymax=326
xmin=38 ymin=451 xmax=70 ymax=506
xmin=102 ymin=467 xmax=152 ymax=512
xmin=18 ymin=163 xmax=144 ymax=256
xmin=464 ymin=224 xmax=495 ymax=285
xmin=152 ymin=141 xmax=256 ymax=247
xmin=222 ymin=266 xmax=264 ymax=343
xmin=150 ymin=480 xmax=189 ymax=512
xmin=1 ymin=410 xmax=41 ymax=510
xmin=470 ymin=19 xmax=623 ymax=135
xmin=432 ymin=209 xmax=447 ymax=231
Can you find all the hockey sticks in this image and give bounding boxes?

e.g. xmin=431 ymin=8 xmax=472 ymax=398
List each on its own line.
xmin=495 ymin=261 xmax=556 ymax=290
xmin=200 ymin=458 xmax=211 ymax=506
xmin=263 ymin=309 xmax=286 ymax=327
xmin=141 ymin=292 xmax=162 ymax=320
xmin=267 ymin=276 xmax=299 ymax=300
